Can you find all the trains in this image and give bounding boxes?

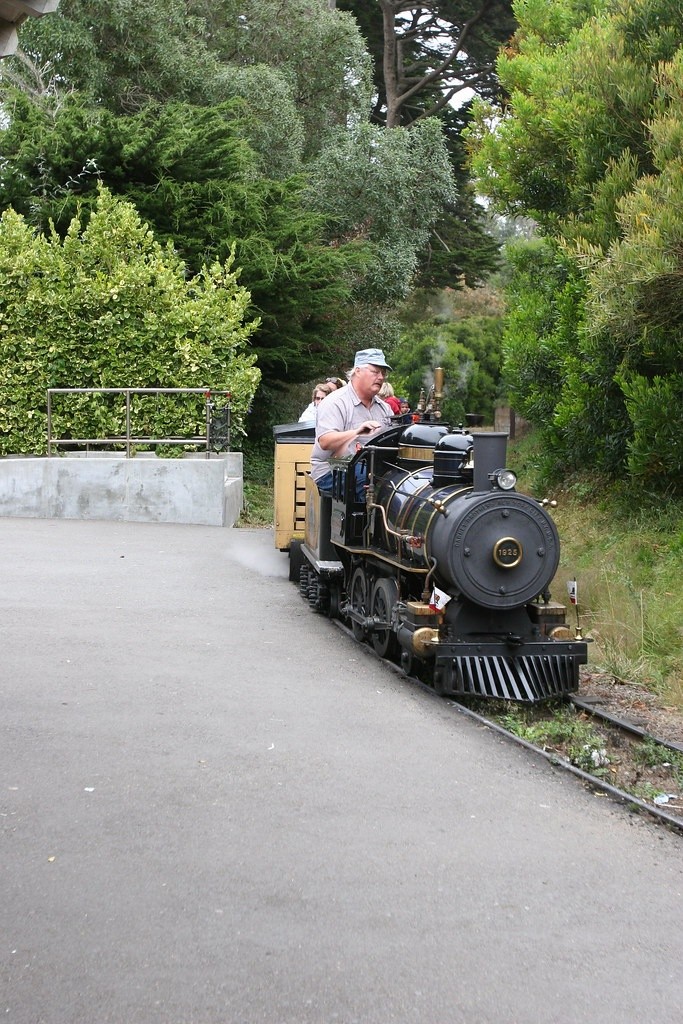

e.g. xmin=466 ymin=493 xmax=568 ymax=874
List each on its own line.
xmin=272 ymin=419 xmax=594 ymax=703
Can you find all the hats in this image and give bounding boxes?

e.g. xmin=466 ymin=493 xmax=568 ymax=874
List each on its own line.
xmin=399 ymin=397 xmax=408 ymax=402
xmin=354 ymin=349 xmax=393 ymax=372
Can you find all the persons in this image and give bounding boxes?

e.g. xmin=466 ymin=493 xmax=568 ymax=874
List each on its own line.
xmin=298 ymin=375 xmax=437 ymax=425
xmin=310 ymin=349 xmax=398 ymax=503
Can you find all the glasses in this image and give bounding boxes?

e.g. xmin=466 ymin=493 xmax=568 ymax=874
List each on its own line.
xmin=315 ymin=397 xmax=324 ymax=401
xmin=325 ymin=377 xmax=338 ymax=384
